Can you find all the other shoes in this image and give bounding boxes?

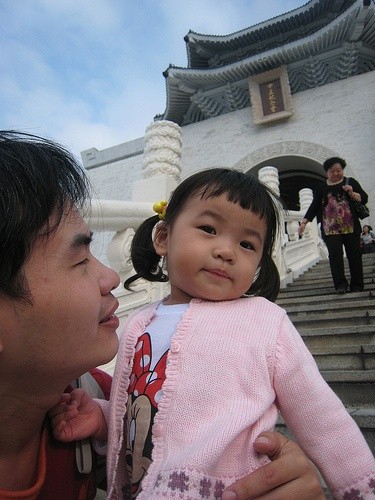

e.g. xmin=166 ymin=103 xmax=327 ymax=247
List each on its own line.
xmin=351 ymin=289 xmax=362 ymax=293
xmin=337 ymin=287 xmax=346 ymax=294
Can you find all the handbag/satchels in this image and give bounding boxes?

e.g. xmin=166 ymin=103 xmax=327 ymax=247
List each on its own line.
xmin=345 ymin=176 xmax=370 ymax=220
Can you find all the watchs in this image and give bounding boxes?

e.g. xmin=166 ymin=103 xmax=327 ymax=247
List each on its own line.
xmin=300 ymin=221 xmax=306 ymax=224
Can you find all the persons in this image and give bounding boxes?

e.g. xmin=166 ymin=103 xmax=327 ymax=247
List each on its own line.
xmin=297 ymin=157 xmax=369 ymax=294
xmin=48 ymin=167 xmax=375 ymax=500
xmin=0 ymin=129 xmax=325 ymax=500
xmin=360 ymin=225 xmax=374 ymax=254
